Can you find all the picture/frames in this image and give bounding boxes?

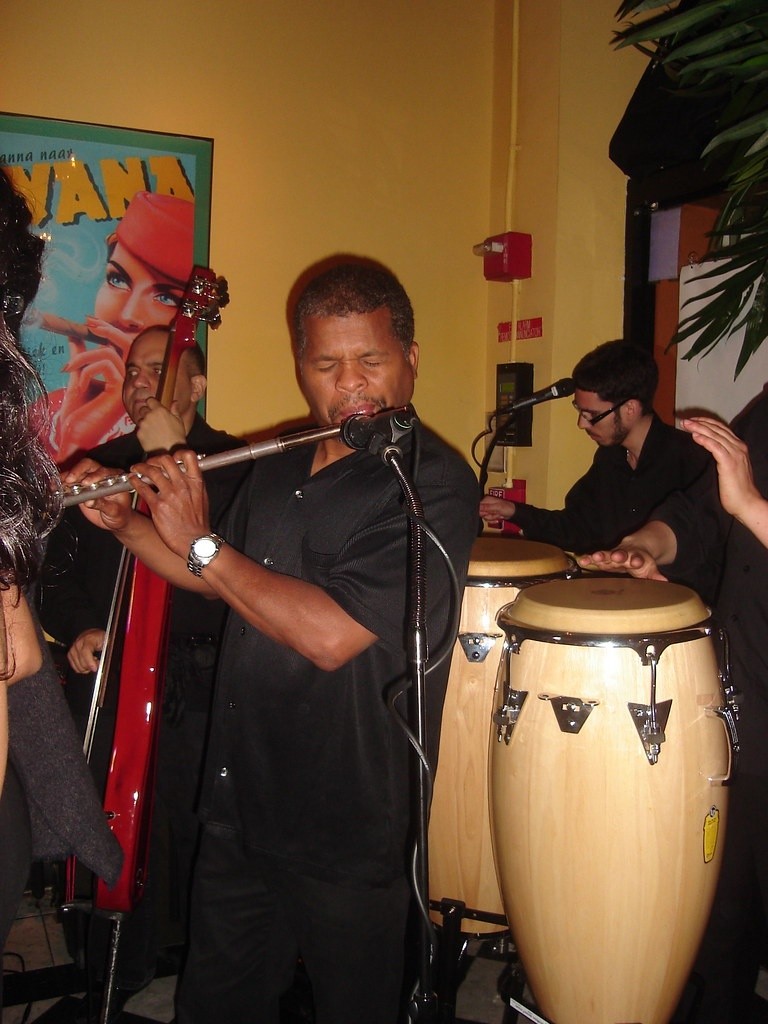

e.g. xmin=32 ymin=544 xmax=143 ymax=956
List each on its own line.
xmin=0 ymin=109 xmax=215 ymax=462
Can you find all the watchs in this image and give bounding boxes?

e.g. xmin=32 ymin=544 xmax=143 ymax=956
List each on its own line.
xmin=186 ymin=533 xmax=225 ymax=578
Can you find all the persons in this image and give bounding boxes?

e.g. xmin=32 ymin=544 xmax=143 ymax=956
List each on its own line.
xmin=578 ymin=384 xmax=768 ymax=1024
xmin=479 ymin=341 xmax=709 ymax=553
xmin=0 ymin=163 xmax=481 ymax=1024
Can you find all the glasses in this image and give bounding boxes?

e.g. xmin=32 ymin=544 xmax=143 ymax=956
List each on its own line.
xmin=572 ymin=399 xmax=629 ymax=426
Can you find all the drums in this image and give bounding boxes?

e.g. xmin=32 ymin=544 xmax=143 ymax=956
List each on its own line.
xmin=426 ymin=529 xmax=577 ymax=954
xmin=493 ymin=575 xmax=734 ymax=1024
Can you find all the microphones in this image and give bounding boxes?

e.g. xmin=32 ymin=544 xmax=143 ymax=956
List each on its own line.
xmin=494 ymin=378 xmax=575 ymax=416
xmin=340 ymin=406 xmax=419 ymax=449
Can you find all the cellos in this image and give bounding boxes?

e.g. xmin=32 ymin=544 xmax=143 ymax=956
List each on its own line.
xmin=62 ymin=264 xmax=231 ymax=1024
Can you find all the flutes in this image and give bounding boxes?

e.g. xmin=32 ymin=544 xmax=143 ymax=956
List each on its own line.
xmin=49 ymin=400 xmax=416 ymax=507
